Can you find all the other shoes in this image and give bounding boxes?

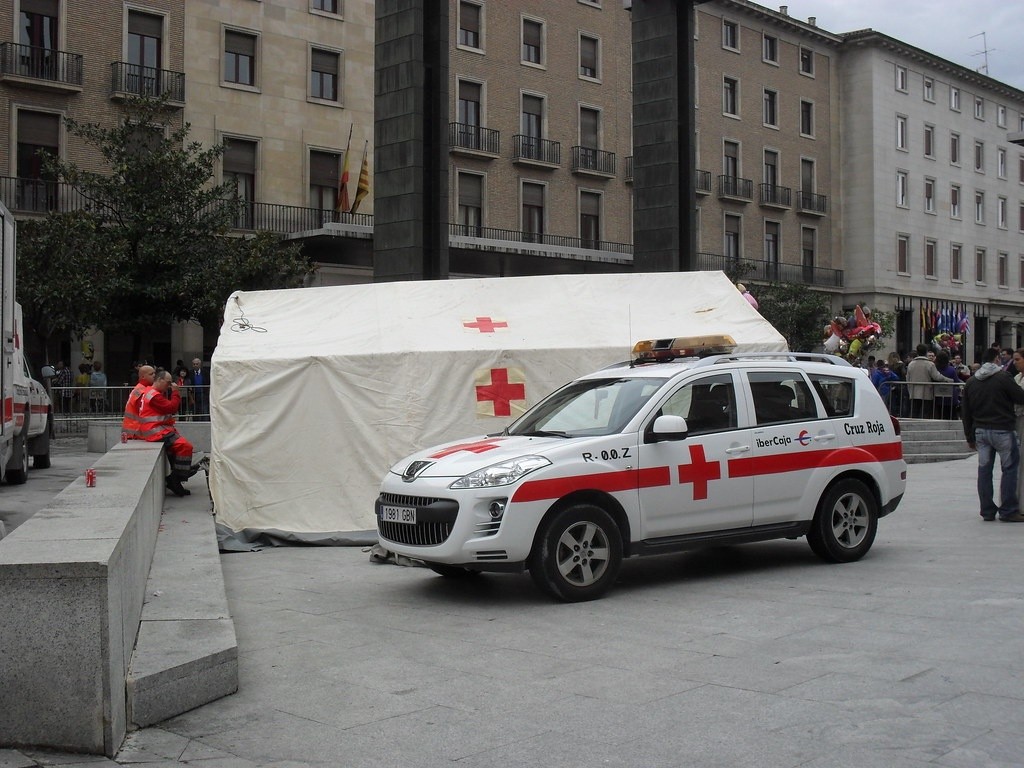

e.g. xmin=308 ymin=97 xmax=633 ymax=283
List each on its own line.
xmin=166 ymin=474 xmax=192 ymax=497
xmin=983 ymin=514 xmax=996 ymax=521
xmin=999 ymin=512 xmax=1024 ymax=522
xmin=188 ymin=463 xmax=199 ymax=478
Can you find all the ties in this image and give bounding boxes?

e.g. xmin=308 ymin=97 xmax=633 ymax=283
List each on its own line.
xmin=196 ymin=371 xmax=198 ymax=375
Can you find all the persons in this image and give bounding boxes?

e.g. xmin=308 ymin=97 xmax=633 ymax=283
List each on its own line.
xmin=138 ymin=371 xmax=193 ymax=497
xmin=1012 ymin=350 xmax=1024 ymax=516
xmin=122 ymin=365 xmax=199 ymax=482
xmin=52 ymin=360 xmax=107 ymax=413
xmin=854 ymin=343 xmax=1019 ymax=421
xmin=960 ymin=348 xmax=1024 ymax=522
xmin=125 ymin=358 xmax=208 ymax=421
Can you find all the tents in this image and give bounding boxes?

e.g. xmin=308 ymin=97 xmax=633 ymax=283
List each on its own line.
xmin=209 ymin=271 xmax=788 ymax=552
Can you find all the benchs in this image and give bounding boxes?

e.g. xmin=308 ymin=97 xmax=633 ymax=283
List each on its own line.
xmin=752 ymin=385 xmax=802 ymax=424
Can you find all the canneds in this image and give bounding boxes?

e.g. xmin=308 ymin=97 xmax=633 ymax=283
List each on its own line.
xmin=121 ymin=431 xmax=129 ymax=444
xmin=86 ymin=468 xmax=97 ymax=487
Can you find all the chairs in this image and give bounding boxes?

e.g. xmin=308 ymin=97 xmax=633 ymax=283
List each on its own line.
xmin=615 ymin=397 xmax=663 ymax=434
xmin=690 ymin=393 xmax=730 ymax=432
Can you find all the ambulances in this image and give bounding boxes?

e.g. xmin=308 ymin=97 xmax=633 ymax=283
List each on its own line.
xmin=0 ymin=202 xmax=58 ymax=485
xmin=374 ymin=339 xmax=910 ymax=603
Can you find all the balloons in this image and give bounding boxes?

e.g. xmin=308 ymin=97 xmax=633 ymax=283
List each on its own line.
xmin=823 ymin=305 xmax=881 ymax=367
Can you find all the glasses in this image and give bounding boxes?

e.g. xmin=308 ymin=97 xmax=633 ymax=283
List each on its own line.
xmin=162 ymin=377 xmax=171 ymax=385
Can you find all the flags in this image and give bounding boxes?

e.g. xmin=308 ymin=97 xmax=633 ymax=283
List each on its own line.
xmin=920 ymin=300 xmax=970 ymax=334
